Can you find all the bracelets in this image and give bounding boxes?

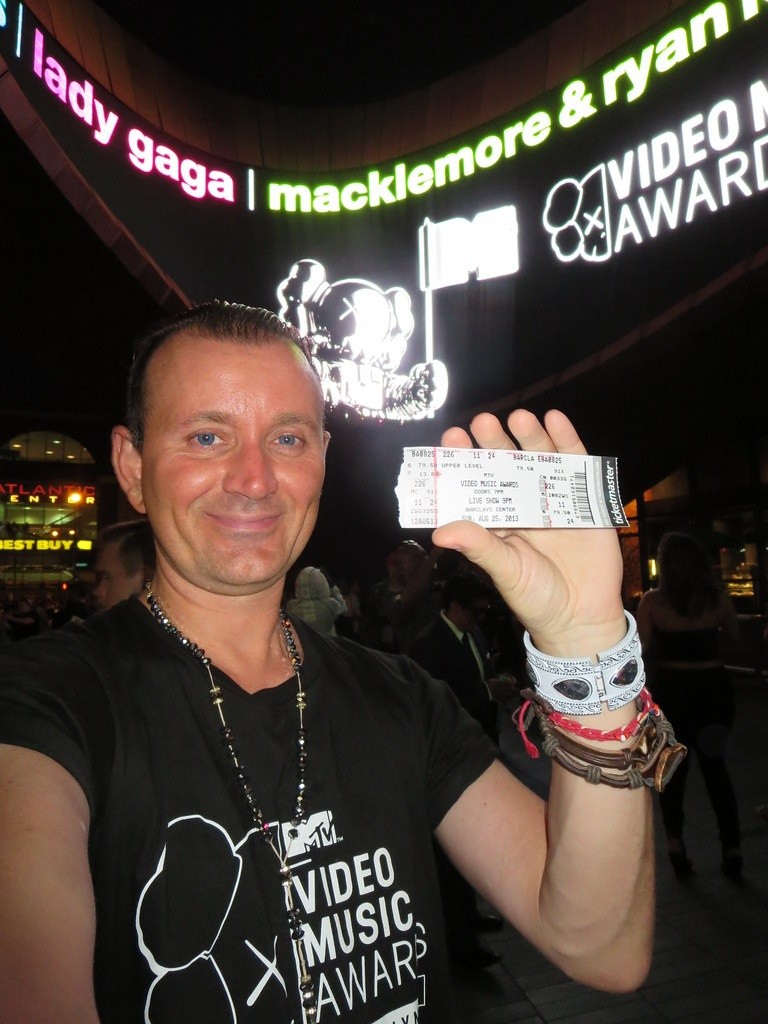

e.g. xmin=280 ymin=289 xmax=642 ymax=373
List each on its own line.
xmin=512 ymin=609 xmax=686 ymax=792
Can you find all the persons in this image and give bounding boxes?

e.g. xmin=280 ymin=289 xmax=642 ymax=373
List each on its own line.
xmin=0 ymin=301 xmax=657 ymax=1024
xmin=639 ymin=531 xmax=745 ymax=882
xmin=92 ymin=520 xmax=158 ymax=615
xmin=0 ymin=578 xmax=92 ymax=646
xmin=286 ymin=538 xmax=537 ymax=972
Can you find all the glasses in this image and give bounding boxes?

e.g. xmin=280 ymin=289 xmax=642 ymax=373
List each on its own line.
xmin=466 ymin=605 xmax=490 ymax=617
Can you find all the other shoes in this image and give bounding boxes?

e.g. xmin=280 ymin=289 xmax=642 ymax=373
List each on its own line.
xmin=720 ymin=852 xmax=744 ymax=876
xmin=668 ymin=837 xmax=692 ymax=873
xmin=449 ymin=946 xmax=500 ymax=967
xmin=467 ymin=913 xmax=502 ymax=938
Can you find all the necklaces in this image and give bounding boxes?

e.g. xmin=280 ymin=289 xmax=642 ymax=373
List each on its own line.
xmin=145 ymin=582 xmax=317 ymax=1024
